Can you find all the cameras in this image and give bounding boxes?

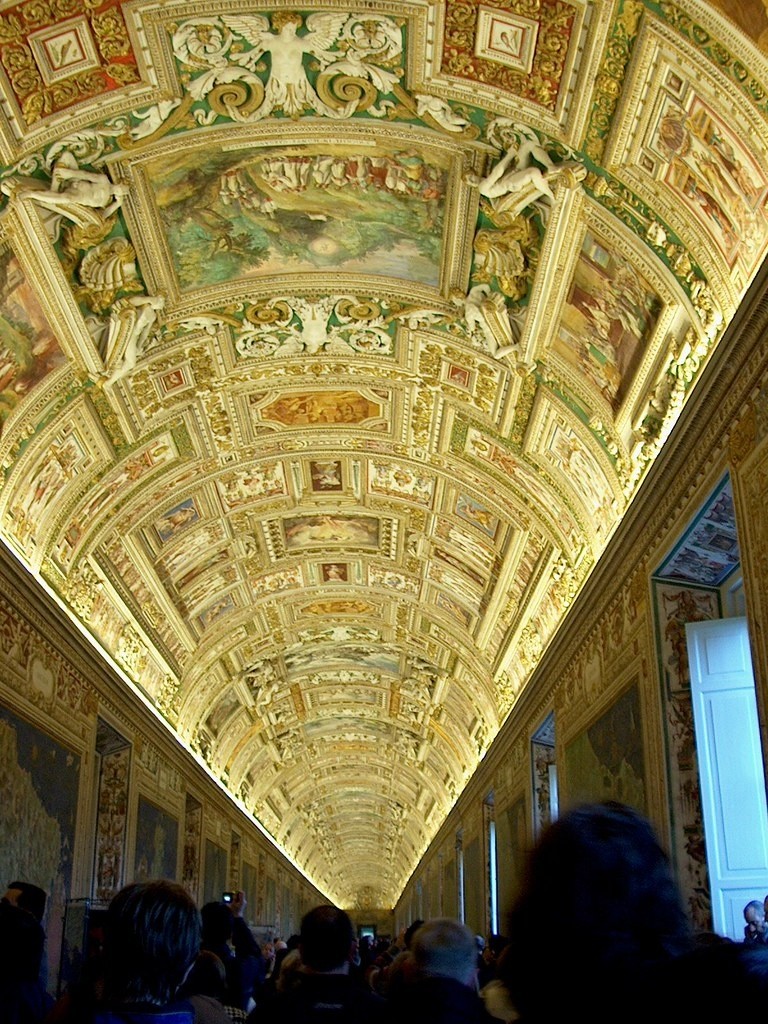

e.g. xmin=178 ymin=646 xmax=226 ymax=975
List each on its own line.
xmin=223 ymin=892 xmax=236 ymax=904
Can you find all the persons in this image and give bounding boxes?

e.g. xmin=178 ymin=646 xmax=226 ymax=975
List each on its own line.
xmin=0 ymin=800 xmax=768 ymax=1024
xmin=56 ymin=882 xmax=235 ymax=1024
